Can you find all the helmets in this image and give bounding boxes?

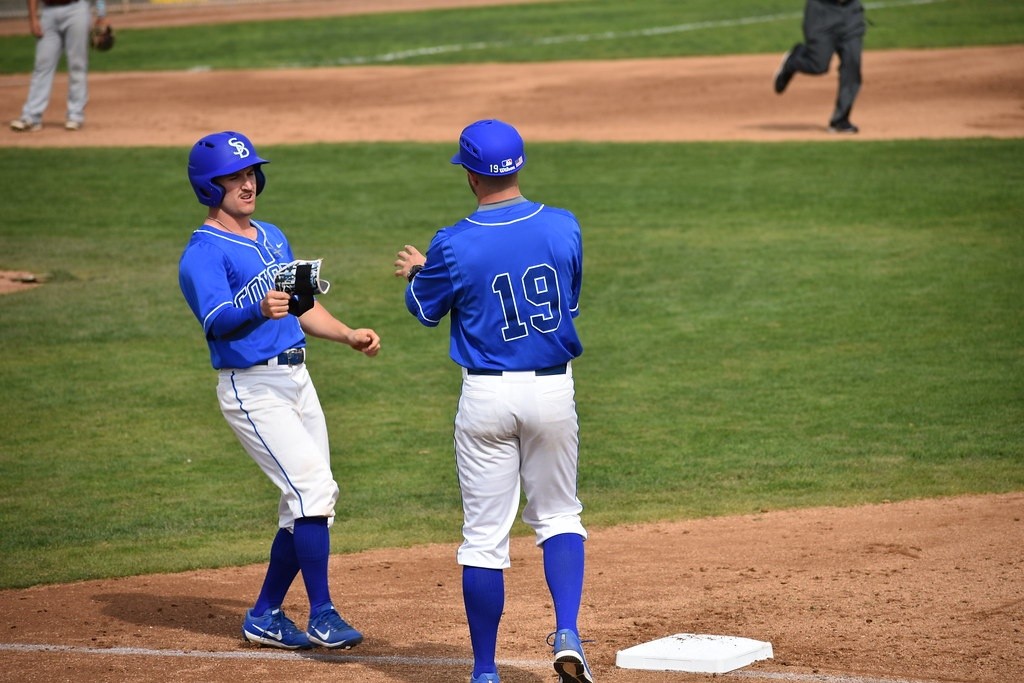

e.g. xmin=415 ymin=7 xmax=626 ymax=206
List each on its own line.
xmin=189 ymin=131 xmax=271 ymax=207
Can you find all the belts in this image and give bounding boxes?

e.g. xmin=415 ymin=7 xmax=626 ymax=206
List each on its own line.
xmin=257 ymin=351 xmax=304 ymax=365
xmin=468 ymin=361 xmax=568 ymax=377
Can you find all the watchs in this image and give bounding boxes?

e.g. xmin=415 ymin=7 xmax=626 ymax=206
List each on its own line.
xmin=406 ymin=265 xmax=421 ymax=283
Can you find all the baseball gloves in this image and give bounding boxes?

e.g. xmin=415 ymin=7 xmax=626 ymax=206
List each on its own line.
xmin=91 ymin=24 xmax=115 ymax=52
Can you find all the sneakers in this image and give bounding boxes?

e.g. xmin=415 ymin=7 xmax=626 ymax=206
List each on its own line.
xmin=64 ymin=120 xmax=83 ymax=130
xmin=11 ymin=119 xmax=41 ymax=132
xmin=471 ymin=671 xmax=500 ymax=683
xmin=241 ymin=609 xmax=315 ymax=650
xmin=829 ymin=120 xmax=858 ymax=134
xmin=548 ymin=630 xmax=594 ymax=683
xmin=306 ymin=602 xmax=363 ymax=647
xmin=775 ymin=51 xmax=795 ymax=93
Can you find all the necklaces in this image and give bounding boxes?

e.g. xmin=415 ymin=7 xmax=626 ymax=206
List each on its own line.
xmin=207 ymin=216 xmax=283 ymax=258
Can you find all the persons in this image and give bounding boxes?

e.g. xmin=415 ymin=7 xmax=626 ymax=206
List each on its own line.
xmin=773 ymin=0 xmax=866 ymax=134
xmin=394 ymin=118 xmax=593 ymax=683
xmin=9 ymin=0 xmax=115 ymax=133
xmin=178 ymin=131 xmax=381 ymax=650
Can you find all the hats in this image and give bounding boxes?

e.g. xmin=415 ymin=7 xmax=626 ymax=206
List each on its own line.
xmin=450 ymin=119 xmax=526 ymax=177
